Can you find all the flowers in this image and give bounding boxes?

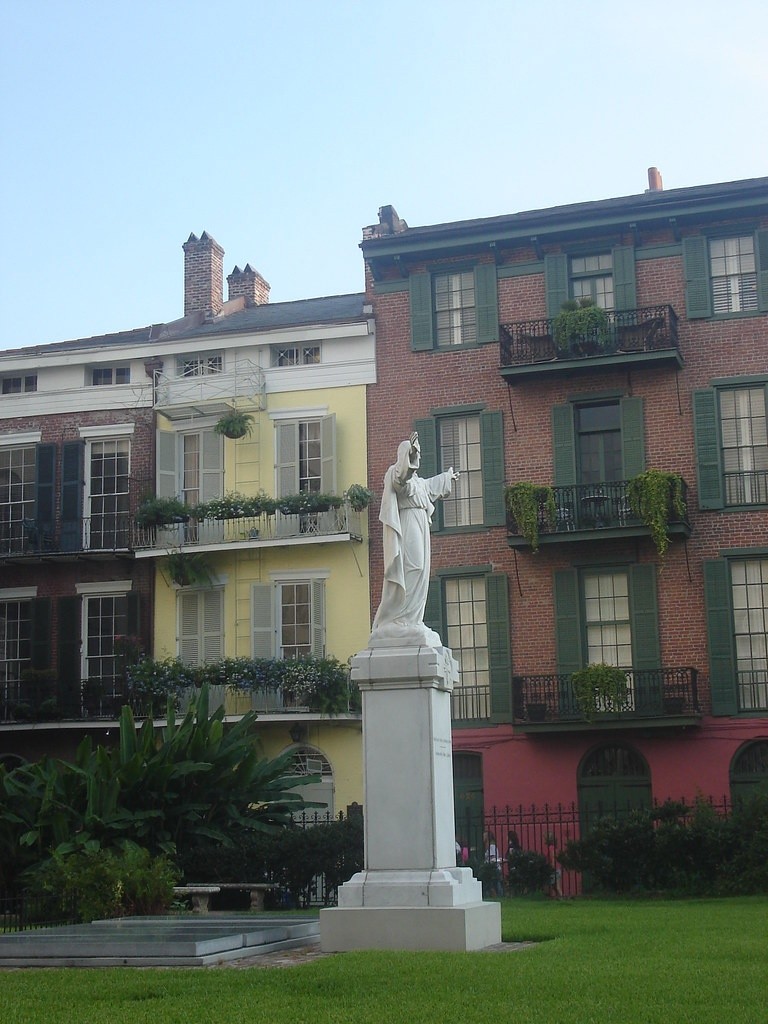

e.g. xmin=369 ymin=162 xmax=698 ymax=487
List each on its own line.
xmin=132 ymin=484 xmax=370 ymax=529
xmin=128 ymin=653 xmax=340 ymax=700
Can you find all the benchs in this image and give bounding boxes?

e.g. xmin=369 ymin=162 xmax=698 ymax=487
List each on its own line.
xmin=186 ymin=883 xmax=280 ymax=911
xmin=174 ymin=887 xmax=221 ymax=913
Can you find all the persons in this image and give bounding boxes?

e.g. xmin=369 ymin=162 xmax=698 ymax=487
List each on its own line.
xmin=370 ymin=431 xmax=461 ymax=644
xmin=455 ymin=829 xmax=563 ymax=899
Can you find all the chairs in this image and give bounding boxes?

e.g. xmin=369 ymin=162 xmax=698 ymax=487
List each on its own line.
xmin=537 ymin=504 xmax=575 ymax=532
xmin=22 ymin=519 xmax=54 ymax=551
xmin=618 ymin=494 xmax=636 ymax=526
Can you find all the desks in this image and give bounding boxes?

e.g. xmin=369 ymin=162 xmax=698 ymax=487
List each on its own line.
xmin=581 ymin=495 xmax=611 ymax=527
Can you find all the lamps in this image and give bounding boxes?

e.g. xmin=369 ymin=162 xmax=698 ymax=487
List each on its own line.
xmin=289 ymin=722 xmax=306 ymax=742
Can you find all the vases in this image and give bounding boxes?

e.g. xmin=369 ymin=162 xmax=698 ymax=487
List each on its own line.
xmin=664 ymin=696 xmax=685 ymax=715
xmin=158 ymin=515 xmax=189 ymax=523
xmin=525 ymin=703 xmax=548 ymax=723
xmin=217 ymin=509 xmax=262 ymax=518
xmin=282 ymin=504 xmax=331 ymax=515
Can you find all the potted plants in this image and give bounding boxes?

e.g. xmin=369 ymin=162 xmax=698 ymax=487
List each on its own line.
xmin=162 ymin=542 xmax=220 ymax=590
xmin=213 ymin=409 xmax=256 ymax=440
xmin=552 ymin=296 xmax=612 ymax=357
